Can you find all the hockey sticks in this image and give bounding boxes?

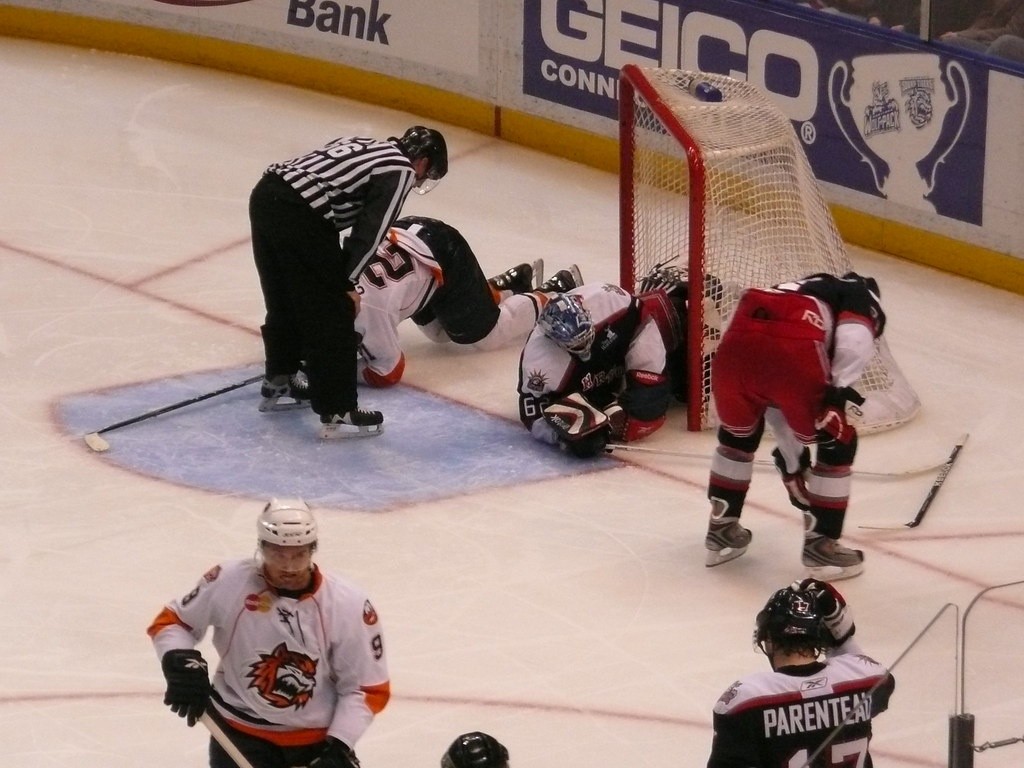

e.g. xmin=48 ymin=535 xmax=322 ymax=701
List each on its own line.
xmin=558 ymin=438 xmax=946 ymax=480
xmin=82 ymin=373 xmax=267 ymax=453
xmin=858 ymin=433 xmax=970 ymax=530
xmin=197 ymin=712 xmax=254 ymax=768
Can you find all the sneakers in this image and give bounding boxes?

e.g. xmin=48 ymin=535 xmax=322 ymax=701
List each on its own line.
xmin=705 ymin=496 xmax=752 ymax=567
xmin=258 ymin=370 xmax=312 ymax=412
xmin=318 ymin=407 xmax=384 ymax=438
xmin=487 ymin=259 xmax=545 ymax=296
xmin=533 ymin=264 xmax=585 ymax=294
xmin=800 ymin=510 xmax=863 ymax=582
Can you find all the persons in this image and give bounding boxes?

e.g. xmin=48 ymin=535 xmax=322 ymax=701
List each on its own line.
xmin=707 ymin=578 xmax=894 ymax=768
xmin=146 ymin=497 xmax=390 ymax=768
xmin=867 ymin=0 xmax=921 ymax=35
xmin=516 ymin=266 xmax=723 ymax=457
xmin=440 ymin=731 xmax=510 ymax=768
xmin=249 ymin=126 xmax=448 ymax=441
xmin=705 ymin=272 xmax=886 ymax=582
xmin=937 ymin=0 xmax=1024 ymax=65
xmin=351 ymin=214 xmax=585 ymax=389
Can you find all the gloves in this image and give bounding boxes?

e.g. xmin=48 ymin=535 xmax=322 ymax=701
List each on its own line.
xmin=771 ymin=446 xmax=812 ymax=510
xmin=161 ymin=649 xmax=211 ymax=727
xmin=295 ymin=741 xmax=346 ymax=768
xmin=814 ymin=384 xmax=866 ymax=449
xmin=796 ymin=577 xmax=856 ymax=644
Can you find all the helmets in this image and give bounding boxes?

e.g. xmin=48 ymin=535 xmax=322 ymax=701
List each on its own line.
xmin=400 ymin=125 xmax=447 ymax=181
xmin=536 ymin=291 xmax=595 ymax=361
xmin=440 ymin=731 xmax=508 ymax=768
xmin=754 ymin=587 xmax=825 ymax=644
xmin=256 ymin=495 xmax=318 ymax=552
